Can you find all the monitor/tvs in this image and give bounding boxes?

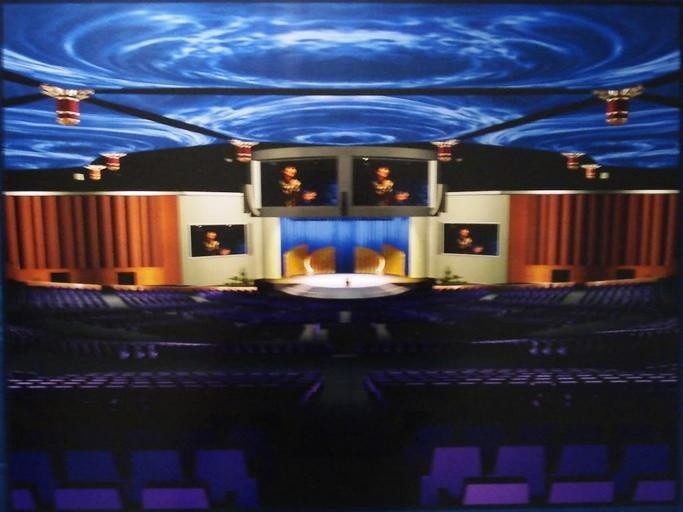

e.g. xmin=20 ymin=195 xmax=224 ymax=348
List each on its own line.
xmin=250 ymin=146 xmax=345 ymax=217
xmin=345 ymin=146 xmax=437 ymax=217
xmin=191 ymin=224 xmax=245 ymax=257
xmin=443 ymin=223 xmax=500 ymax=255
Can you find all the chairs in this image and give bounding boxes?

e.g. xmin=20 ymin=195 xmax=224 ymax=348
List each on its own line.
xmin=6 ymin=283 xmax=679 ymax=511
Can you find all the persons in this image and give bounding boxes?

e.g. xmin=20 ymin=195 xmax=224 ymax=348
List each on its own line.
xmin=199 ymin=229 xmax=232 ymax=254
xmin=364 ymin=163 xmax=410 ymax=206
xmin=450 ymin=226 xmax=483 ymax=253
xmin=270 ymin=163 xmax=318 ymax=208
